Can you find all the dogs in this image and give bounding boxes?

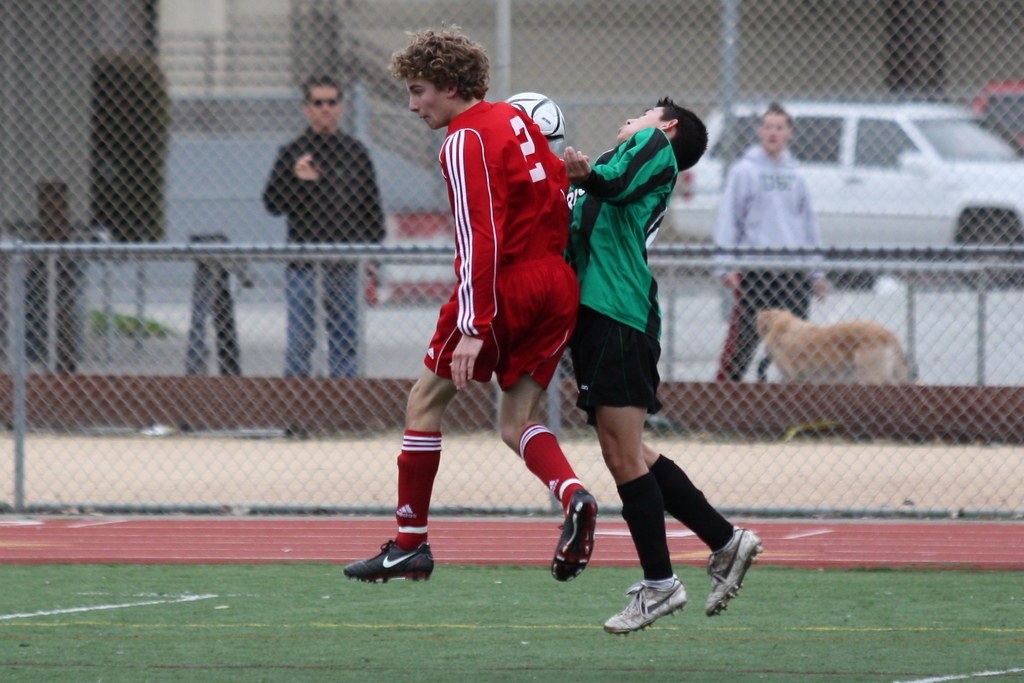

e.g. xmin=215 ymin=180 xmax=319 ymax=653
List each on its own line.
xmin=751 ymin=310 xmax=913 ymax=388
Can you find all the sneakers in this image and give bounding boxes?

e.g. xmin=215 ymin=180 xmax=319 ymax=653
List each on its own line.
xmin=705 ymin=524 xmax=765 ymax=616
xmin=603 ymin=574 xmax=688 ymax=637
xmin=551 ymin=491 xmax=598 ymax=582
xmin=344 ymin=539 xmax=435 ymax=583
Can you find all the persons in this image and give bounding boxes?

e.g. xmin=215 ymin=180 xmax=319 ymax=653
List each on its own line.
xmin=558 ymin=96 xmax=764 ymax=636
xmin=714 ymin=101 xmax=826 ymax=382
xmin=263 ymin=75 xmax=388 ymax=378
xmin=343 ymin=29 xmax=599 ymax=584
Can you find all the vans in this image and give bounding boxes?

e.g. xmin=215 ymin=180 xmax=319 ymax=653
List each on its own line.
xmin=665 ymin=99 xmax=1024 ymax=289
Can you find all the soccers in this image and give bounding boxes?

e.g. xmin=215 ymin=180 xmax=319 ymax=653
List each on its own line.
xmin=504 ymin=91 xmax=567 ymax=157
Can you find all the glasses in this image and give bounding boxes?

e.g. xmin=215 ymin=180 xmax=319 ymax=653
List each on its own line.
xmin=310 ymin=99 xmax=340 ymax=108
